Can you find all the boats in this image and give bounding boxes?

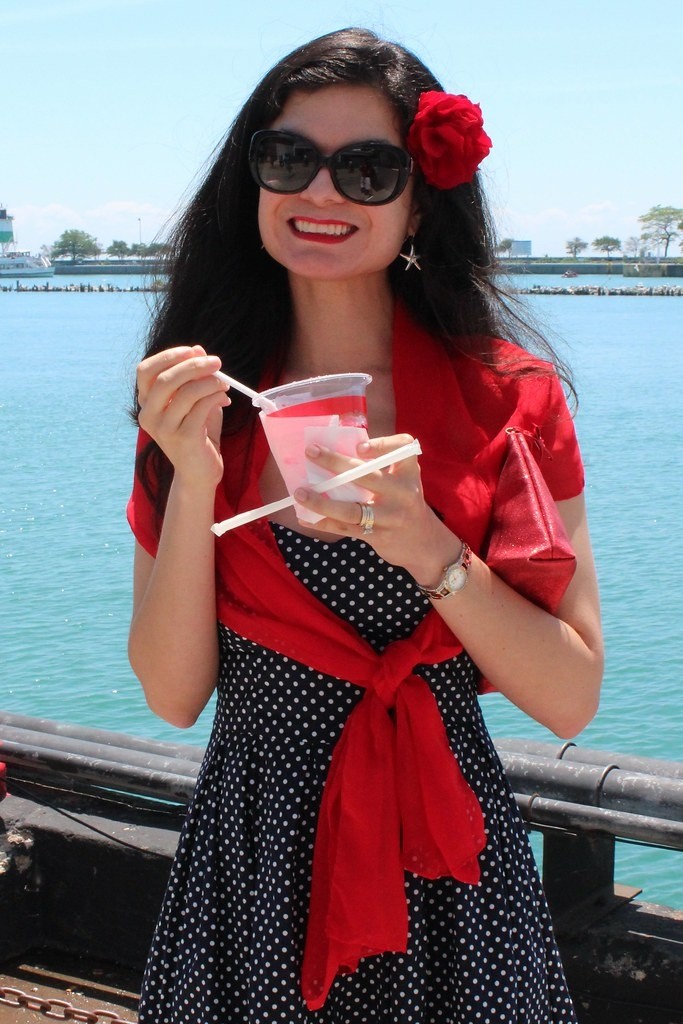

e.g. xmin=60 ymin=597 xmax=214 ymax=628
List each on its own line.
xmin=0 ymin=251 xmax=56 ymax=278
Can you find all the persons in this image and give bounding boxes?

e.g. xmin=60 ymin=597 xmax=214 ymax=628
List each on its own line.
xmin=126 ymin=28 xmax=603 ymax=1024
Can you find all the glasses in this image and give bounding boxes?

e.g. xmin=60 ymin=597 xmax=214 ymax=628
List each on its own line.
xmin=249 ymin=127 xmax=414 ymax=205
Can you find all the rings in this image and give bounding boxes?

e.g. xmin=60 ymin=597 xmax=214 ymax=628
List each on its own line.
xmin=356 ymin=501 xmax=374 ymax=535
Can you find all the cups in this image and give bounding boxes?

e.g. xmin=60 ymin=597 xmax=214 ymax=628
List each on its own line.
xmin=250 ymin=372 xmax=374 ymax=527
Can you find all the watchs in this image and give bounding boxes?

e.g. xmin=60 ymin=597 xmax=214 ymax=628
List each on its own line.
xmin=416 ymin=539 xmax=472 ymax=600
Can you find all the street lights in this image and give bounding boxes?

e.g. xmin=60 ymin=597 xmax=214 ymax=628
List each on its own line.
xmin=138 ymin=218 xmax=142 ymax=244
